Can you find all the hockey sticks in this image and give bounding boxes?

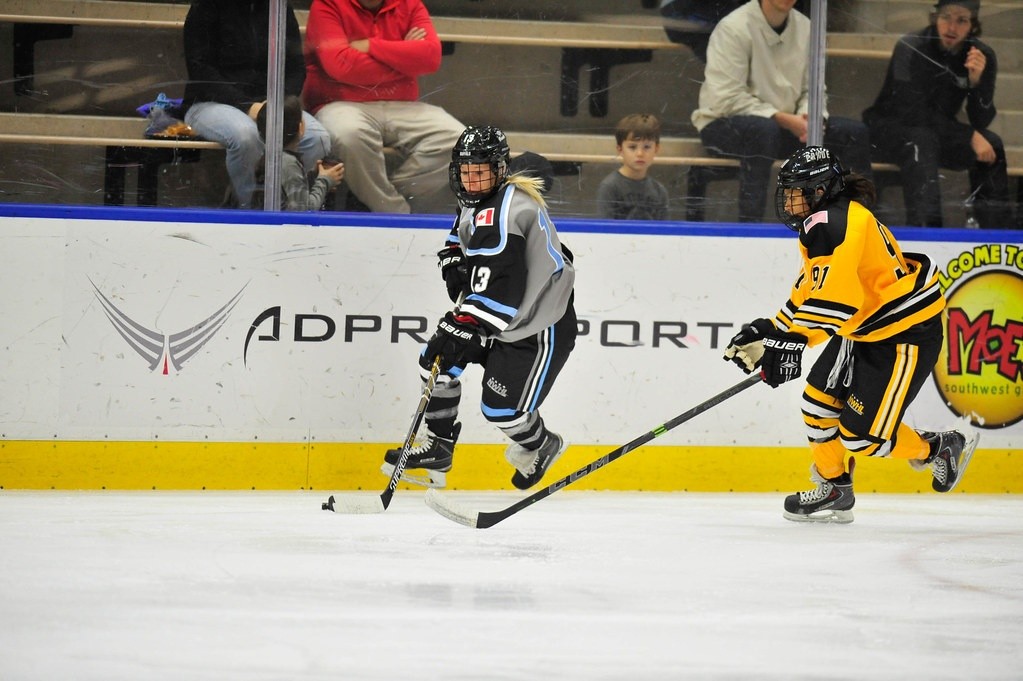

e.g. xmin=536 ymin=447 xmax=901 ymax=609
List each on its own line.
xmin=423 ymin=368 xmax=766 ymax=528
xmin=329 ymin=291 xmax=466 ymax=515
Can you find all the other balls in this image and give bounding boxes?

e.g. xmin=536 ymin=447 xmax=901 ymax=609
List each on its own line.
xmin=322 ymin=503 xmax=329 ymax=509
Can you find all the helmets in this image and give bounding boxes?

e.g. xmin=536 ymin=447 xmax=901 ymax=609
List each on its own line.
xmin=448 ymin=125 xmax=510 ymax=208
xmin=774 ymin=144 xmax=841 ymax=231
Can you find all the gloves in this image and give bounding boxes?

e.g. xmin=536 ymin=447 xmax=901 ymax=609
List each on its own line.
xmin=418 ymin=309 xmax=480 ymax=371
xmin=437 ymin=247 xmax=474 ymax=302
xmin=723 ymin=317 xmax=778 ymax=375
xmin=760 ymin=332 xmax=808 ymax=389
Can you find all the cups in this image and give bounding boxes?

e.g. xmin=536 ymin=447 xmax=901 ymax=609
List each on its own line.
xmin=315 ymin=156 xmax=341 ymax=194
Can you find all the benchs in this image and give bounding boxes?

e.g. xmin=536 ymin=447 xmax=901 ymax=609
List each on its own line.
xmin=0 ymin=0 xmax=1023 ymax=228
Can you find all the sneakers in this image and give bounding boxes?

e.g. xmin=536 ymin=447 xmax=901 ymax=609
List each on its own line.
xmin=504 ymin=418 xmax=567 ymax=491
xmin=784 ymin=455 xmax=856 ymax=523
xmin=908 ymin=428 xmax=980 ymax=493
xmin=381 ymin=421 xmax=461 ymax=488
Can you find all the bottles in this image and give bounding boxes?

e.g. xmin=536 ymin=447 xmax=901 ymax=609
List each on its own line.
xmin=963 ymin=211 xmax=980 ymax=229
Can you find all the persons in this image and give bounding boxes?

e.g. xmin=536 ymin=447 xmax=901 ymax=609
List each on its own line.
xmin=382 ymin=125 xmax=578 ymax=490
xmin=181 ymin=0 xmax=331 ymax=209
xmin=252 ymin=96 xmax=344 ymax=211
xmin=723 ymin=145 xmax=981 ymax=524
xmin=690 ymin=0 xmax=870 ymax=222
xmin=596 ymin=113 xmax=670 ymax=221
xmin=864 ymin=0 xmax=1009 ymax=229
xmin=303 ymin=0 xmax=466 ymax=215
